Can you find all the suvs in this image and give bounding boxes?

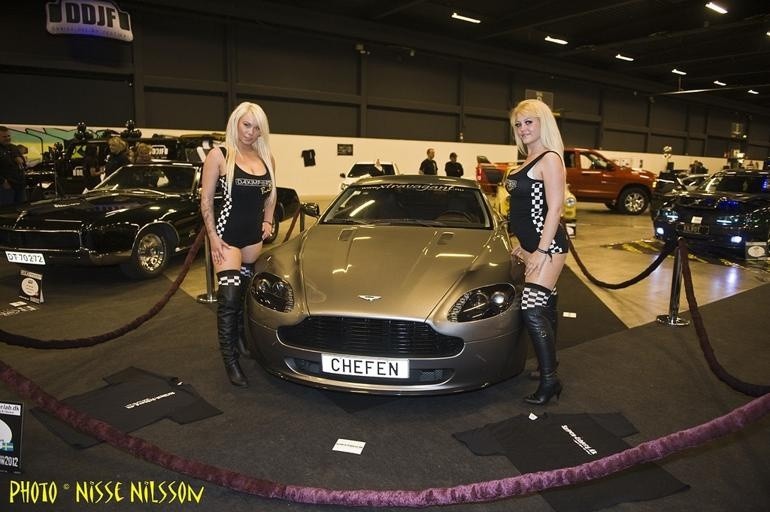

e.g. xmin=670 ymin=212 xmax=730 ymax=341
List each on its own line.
xmin=649 ymin=166 xmax=770 ymax=256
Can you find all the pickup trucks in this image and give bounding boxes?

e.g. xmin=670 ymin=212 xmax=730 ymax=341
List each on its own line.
xmin=473 ymin=146 xmax=659 ymax=215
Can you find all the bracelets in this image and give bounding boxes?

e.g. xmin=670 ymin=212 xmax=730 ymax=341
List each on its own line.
xmin=264 ymin=221 xmax=272 ymax=226
xmin=537 ymin=248 xmax=549 ymax=254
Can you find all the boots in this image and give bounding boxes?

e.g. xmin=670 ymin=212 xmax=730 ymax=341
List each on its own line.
xmin=218 ymin=275 xmax=250 ymax=388
xmin=521 ymin=294 xmax=563 ymax=405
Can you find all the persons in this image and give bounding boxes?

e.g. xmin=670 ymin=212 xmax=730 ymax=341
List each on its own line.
xmin=445 ymin=153 xmax=463 ymax=177
xmin=505 ymin=99 xmax=569 ymax=406
xmin=419 ymin=148 xmax=439 ymax=175
xmin=0 ymin=126 xmax=27 ymax=205
xmin=200 ymin=101 xmax=277 ymax=388
xmin=81 ymin=137 xmax=161 ymax=195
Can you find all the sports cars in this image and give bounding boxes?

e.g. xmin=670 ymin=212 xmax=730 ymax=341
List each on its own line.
xmin=237 ymin=172 xmax=529 ymax=402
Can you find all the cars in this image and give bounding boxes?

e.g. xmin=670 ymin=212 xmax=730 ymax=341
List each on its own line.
xmin=338 ymin=161 xmax=406 ymax=194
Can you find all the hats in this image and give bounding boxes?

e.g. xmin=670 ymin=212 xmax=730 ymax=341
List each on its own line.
xmin=450 ymin=152 xmax=457 ymax=157
